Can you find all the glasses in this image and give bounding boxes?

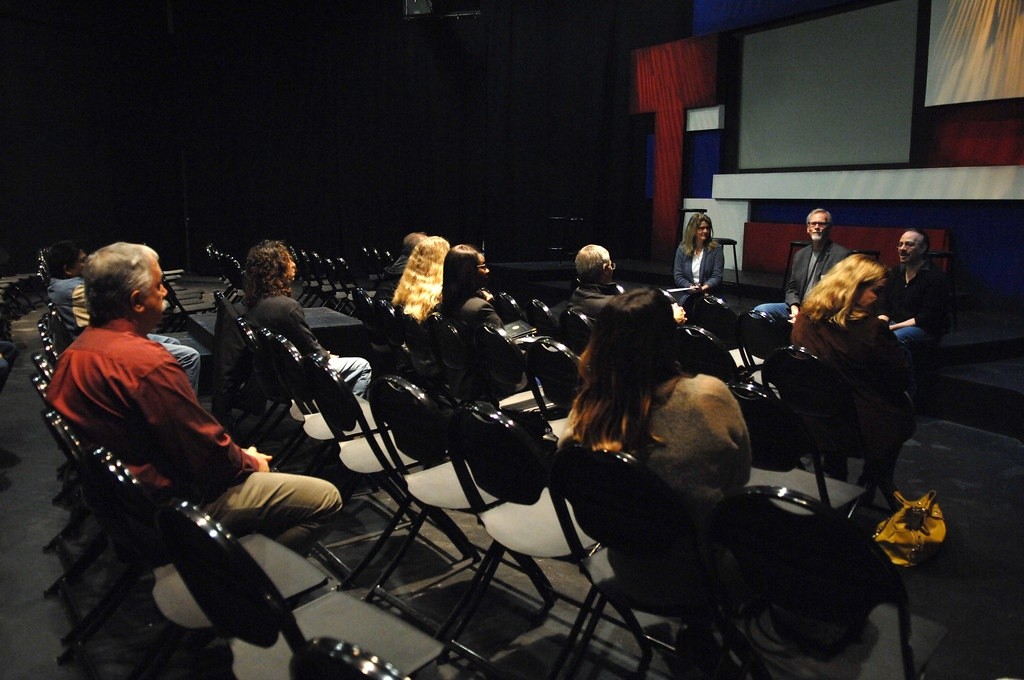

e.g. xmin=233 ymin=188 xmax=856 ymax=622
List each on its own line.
xmin=808 ymin=221 xmax=828 ymax=227
xmin=477 ymin=261 xmax=487 ymax=269
xmin=895 ymin=241 xmax=925 ymax=248
xmin=608 ymin=263 xmax=616 ymax=271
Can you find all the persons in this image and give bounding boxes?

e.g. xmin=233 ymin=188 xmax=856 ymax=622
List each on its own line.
xmin=557 ymin=284 xmax=752 ymax=487
xmin=240 ymin=239 xmax=372 ymax=397
xmin=753 ymin=204 xmax=852 ymax=328
xmin=378 ymin=233 xmax=505 ymax=331
xmin=673 ymin=212 xmax=724 ymax=313
xmin=791 ymin=254 xmax=916 ymax=407
xmin=874 ymin=226 xmax=950 ymax=394
xmin=47 ymin=241 xmax=200 ymax=395
xmin=566 ymin=242 xmax=617 ymax=317
xmin=46 ymin=241 xmax=347 ymax=555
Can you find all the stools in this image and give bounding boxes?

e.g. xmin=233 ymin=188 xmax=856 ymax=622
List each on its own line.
xmin=782 ymin=240 xmax=811 ymax=290
xmin=925 ymin=251 xmax=958 ymax=332
xmin=851 ymin=248 xmax=881 ymax=263
xmin=710 ymin=239 xmax=741 ymax=303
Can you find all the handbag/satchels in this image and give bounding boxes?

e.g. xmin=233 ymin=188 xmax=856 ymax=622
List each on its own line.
xmin=873 ymin=491 xmax=946 ymax=567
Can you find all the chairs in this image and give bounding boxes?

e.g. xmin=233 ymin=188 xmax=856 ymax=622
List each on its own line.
xmin=0 ymin=245 xmax=917 ymax=680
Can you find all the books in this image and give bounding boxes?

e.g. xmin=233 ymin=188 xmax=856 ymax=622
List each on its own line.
xmin=503 ymin=318 xmax=537 ymax=340
